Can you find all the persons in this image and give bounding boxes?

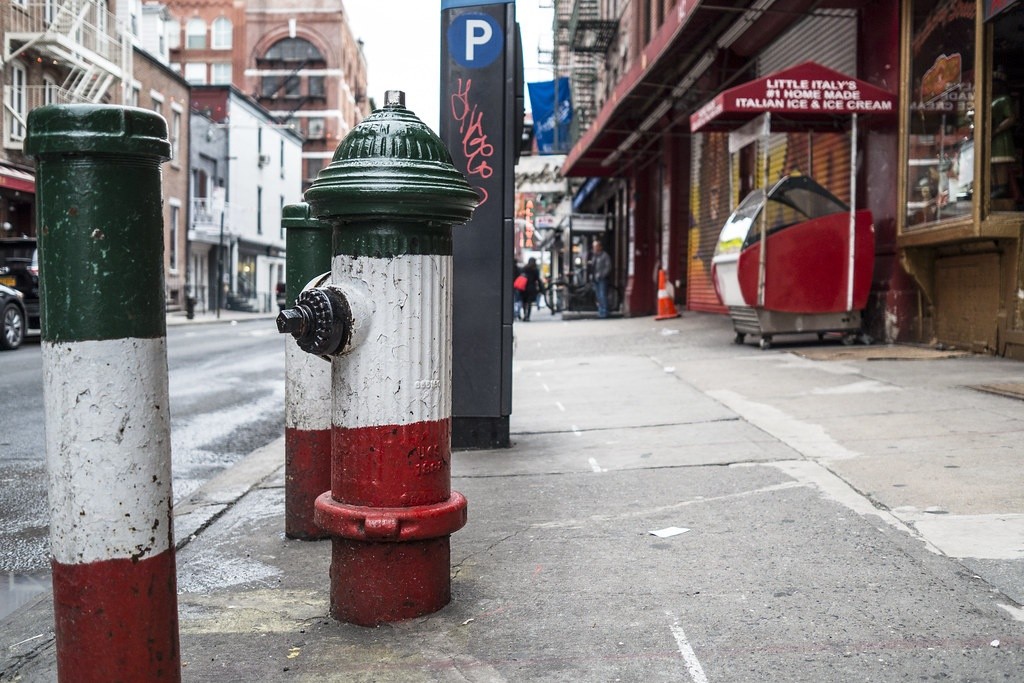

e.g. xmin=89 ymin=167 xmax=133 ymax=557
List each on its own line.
xmin=587 ymin=239 xmax=612 ymax=319
xmin=514 ymin=257 xmax=545 ymax=322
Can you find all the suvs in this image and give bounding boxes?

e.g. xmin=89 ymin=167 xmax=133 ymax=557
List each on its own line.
xmin=0 ymin=233 xmax=42 ymax=323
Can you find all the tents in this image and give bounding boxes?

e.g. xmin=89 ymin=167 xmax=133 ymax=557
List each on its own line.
xmin=690 ymin=58 xmax=899 ymax=311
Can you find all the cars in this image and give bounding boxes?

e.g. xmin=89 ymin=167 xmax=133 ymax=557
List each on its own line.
xmin=0 ymin=283 xmax=30 ymax=351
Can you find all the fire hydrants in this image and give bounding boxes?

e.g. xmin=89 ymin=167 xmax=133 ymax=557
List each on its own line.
xmin=274 ymin=87 xmax=486 ymax=625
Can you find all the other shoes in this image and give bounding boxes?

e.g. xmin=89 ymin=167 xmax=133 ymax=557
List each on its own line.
xmin=522 ymin=317 xmax=530 ymax=321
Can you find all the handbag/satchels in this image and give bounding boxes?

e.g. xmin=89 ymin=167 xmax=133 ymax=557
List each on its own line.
xmin=513 ymin=272 xmax=528 ymax=291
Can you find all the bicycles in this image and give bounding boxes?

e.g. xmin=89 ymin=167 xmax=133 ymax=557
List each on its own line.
xmin=543 ymin=269 xmax=624 ymax=313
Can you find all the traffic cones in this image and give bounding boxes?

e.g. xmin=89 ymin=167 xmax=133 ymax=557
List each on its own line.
xmin=654 ymin=268 xmax=681 ymax=320
